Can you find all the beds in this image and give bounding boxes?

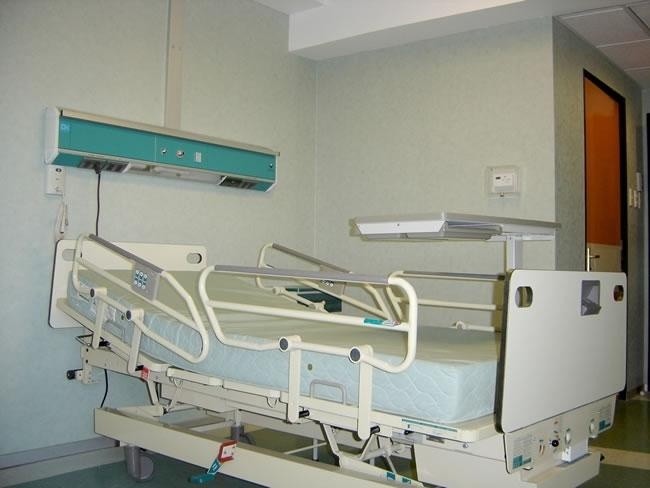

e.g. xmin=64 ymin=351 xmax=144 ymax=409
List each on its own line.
xmin=45 ymin=213 xmax=630 ymax=488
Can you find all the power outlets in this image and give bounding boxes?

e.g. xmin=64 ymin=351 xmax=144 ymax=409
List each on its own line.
xmin=78 ymin=157 xmax=105 ymax=171
xmin=102 ymin=160 xmax=129 ymax=174
